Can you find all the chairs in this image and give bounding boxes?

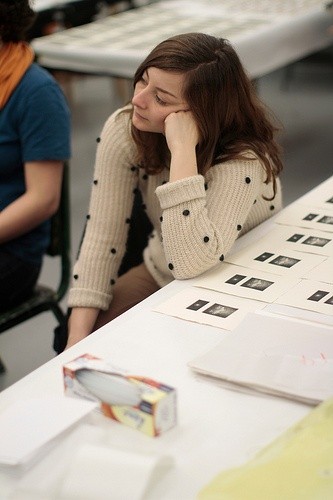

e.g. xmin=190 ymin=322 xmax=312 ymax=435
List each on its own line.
xmin=0 ymin=159 xmax=71 ymax=376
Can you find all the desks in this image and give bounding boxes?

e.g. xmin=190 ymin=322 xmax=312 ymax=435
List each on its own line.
xmin=0 ymin=177 xmax=333 ymax=500
xmin=28 ymin=0 xmax=333 ymax=113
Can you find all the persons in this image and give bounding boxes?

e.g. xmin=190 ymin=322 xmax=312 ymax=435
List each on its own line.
xmin=65 ymin=31 xmax=283 ymax=351
xmin=1 ymin=0 xmax=74 ymax=373
